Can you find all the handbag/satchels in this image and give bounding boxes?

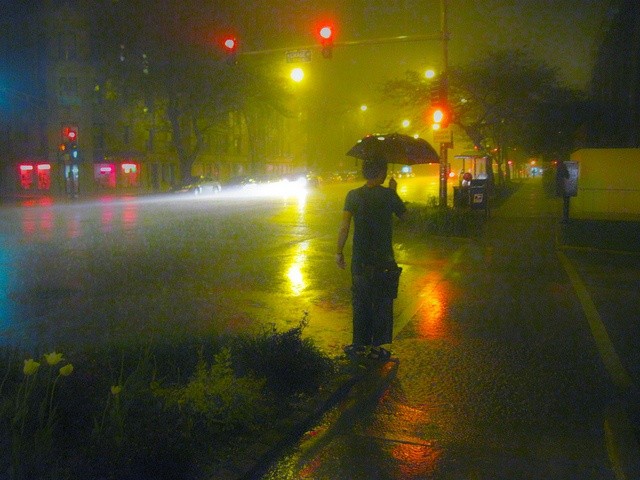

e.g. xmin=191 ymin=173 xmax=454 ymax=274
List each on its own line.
xmin=358 ymin=267 xmax=402 ymax=303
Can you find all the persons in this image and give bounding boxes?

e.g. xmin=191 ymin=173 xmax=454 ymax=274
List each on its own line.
xmin=335 ymin=159 xmax=409 ymax=362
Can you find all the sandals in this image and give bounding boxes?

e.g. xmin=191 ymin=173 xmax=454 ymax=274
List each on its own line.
xmin=368 ymin=348 xmax=391 ymax=360
xmin=344 ymin=345 xmax=367 ymax=356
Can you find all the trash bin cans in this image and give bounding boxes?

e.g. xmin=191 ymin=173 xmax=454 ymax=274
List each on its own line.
xmin=453 ymin=186 xmax=468 ymax=207
xmin=471 ymin=186 xmax=486 ymax=208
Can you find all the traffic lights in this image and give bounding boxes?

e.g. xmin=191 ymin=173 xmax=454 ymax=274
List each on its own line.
xmin=430 ymin=77 xmax=448 ymax=127
xmin=320 ymin=27 xmax=332 ymax=59
xmin=225 ymin=39 xmax=236 ymax=65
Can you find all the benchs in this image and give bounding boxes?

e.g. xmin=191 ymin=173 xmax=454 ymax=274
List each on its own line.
xmin=446 ymin=203 xmax=477 ymax=234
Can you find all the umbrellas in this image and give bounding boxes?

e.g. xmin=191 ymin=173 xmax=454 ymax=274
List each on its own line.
xmin=345 ymin=133 xmax=442 ymax=181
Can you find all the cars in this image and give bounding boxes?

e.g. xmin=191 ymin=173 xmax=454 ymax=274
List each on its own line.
xmin=226 ymin=169 xmax=360 ymax=191
xmin=169 ymin=175 xmax=223 ymax=195
